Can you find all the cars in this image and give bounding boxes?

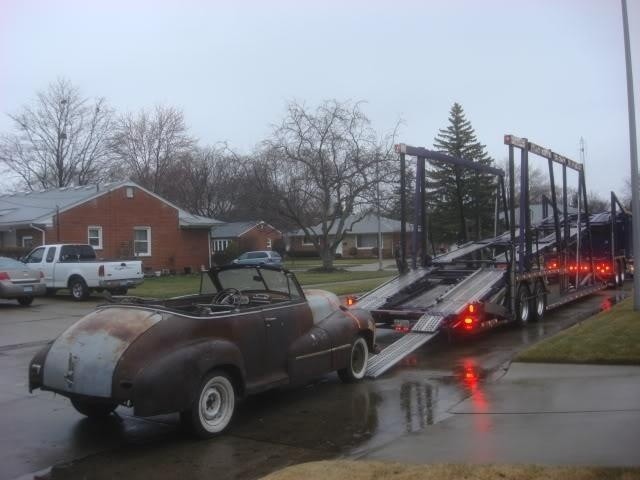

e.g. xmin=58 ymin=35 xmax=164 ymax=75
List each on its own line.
xmin=230 ymin=249 xmax=281 ymax=266
xmin=0 ymin=256 xmax=47 ymax=307
xmin=24 ymin=253 xmax=381 ymax=436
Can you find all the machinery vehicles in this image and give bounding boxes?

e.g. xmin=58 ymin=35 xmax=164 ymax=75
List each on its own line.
xmin=342 ymin=136 xmax=634 ymax=378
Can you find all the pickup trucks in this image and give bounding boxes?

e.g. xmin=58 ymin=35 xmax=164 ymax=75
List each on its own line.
xmin=20 ymin=242 xmax=146 ymax=300
